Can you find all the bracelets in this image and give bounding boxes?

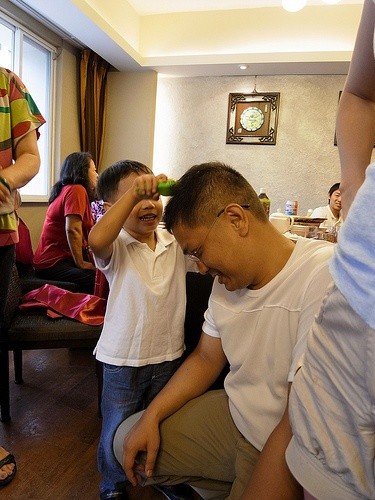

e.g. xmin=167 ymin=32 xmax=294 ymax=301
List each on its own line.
xmin=0 ymin=176 xmax=11 ymax=192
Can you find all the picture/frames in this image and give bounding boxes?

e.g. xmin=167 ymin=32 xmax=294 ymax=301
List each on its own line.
xmin=225 ymin=92 xmax=280 ymax=145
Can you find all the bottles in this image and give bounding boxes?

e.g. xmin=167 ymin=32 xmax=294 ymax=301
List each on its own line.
xmin=258 ymin=188 xmax=271 ymax=221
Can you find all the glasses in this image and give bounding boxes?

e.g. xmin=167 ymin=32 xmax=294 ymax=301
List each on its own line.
xmin=189 ymin=202 xmax=250 ymax=262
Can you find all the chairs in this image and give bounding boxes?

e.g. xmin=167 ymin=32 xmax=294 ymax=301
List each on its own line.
xmin=14 ymin=218 xmax=79 ymax=294
xmin=0 ymin=244 xmax=103 ymax=425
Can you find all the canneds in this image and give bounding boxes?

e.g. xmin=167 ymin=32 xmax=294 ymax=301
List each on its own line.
xmin=285 ymin=200 xmax=298 ymax=215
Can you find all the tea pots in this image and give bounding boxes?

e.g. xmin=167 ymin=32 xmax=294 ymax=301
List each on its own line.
xmin=269 ymin=209 xmax=294 ymax=234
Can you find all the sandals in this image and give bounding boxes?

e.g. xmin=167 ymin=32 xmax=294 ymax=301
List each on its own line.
xmin=0 ymin=446 xmax=17 ymax=489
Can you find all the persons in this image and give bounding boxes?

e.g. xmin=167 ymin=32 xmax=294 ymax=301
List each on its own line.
xmin=114 ymin=162 xmax=336 ymax=500
xmin=0 ymin=67 xmax=46 ymax=486
xmin=88 ymin=159 xmax=200 ymax=500
xmin=285 ymin=0 xmax=375 ymax=500
xmin=32 ymin=152 xmax=99 ymax=350
xmin=307 ymin=183 xmax=341 ymax=237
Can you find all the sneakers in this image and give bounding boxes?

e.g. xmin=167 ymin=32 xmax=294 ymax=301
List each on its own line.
xmin=149 ymin=482 xmax=196 ymax=500
xmin=101 ymin=489 xmax=130 ymax=500
